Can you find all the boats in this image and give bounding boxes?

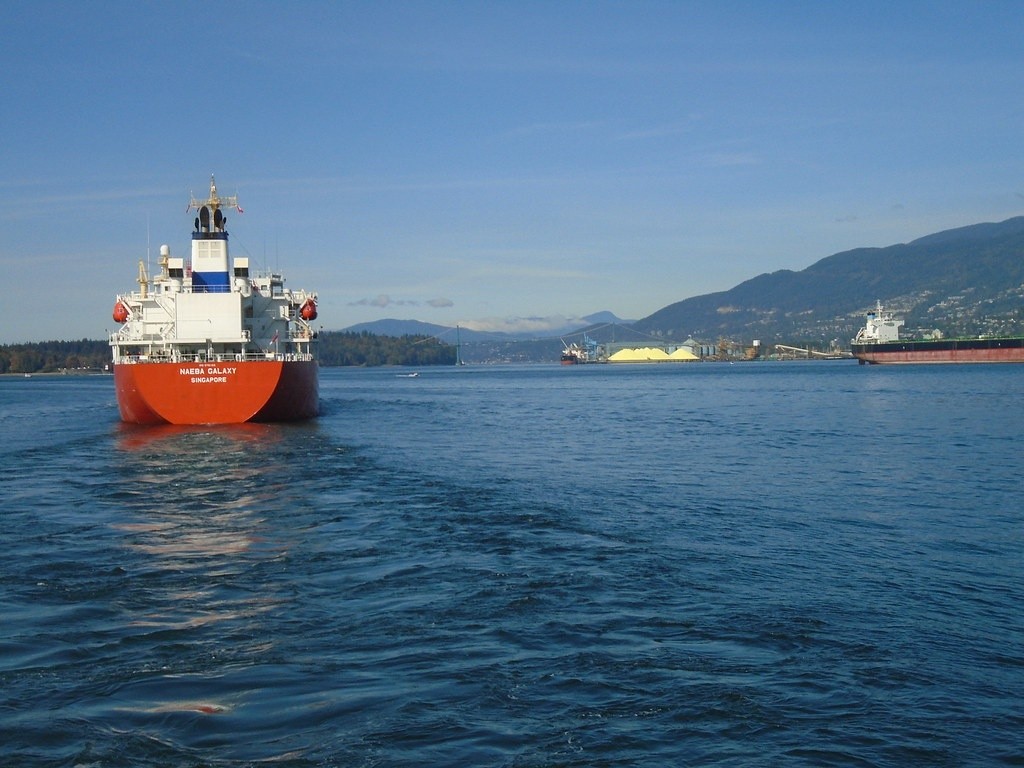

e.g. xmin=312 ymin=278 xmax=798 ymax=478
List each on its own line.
xmin=105 ymin=172 xmax=322 ymax=425
xmin=852 ymin=299 xmax=1024 ymax=365
